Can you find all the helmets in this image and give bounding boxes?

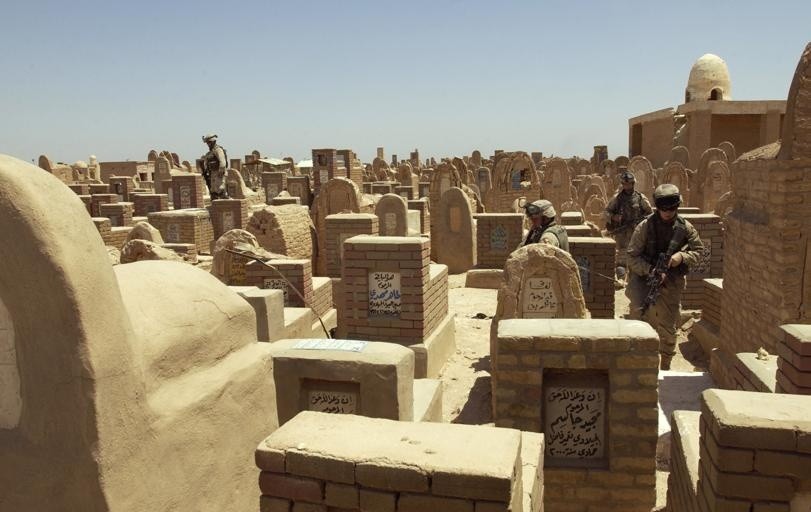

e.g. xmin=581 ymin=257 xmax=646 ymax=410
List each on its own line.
xmin=526 ymin=199 xmax=556 ymax=218
xmin=654 ymin=183 xmax=680 ymax=204
xmin=621 ymin=172 xmax=634 ymax=183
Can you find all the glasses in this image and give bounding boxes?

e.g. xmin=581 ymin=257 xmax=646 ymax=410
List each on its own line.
xmin=656 ymin=204 xmax=679 ymax=212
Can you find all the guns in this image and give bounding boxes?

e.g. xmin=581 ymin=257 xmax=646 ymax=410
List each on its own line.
xmin=199 ymin=160 xmax=211 ymax=190
xmin=605 ymin=217 xmax=647 ymax=232
xmin=641 ymin=225 xmax=689 ymax=316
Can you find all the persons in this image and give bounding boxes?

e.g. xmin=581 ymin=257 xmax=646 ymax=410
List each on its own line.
xmin=603 ymin=172 xmax=652 ymax=279
xmin=624 ymin=184 xmax=705 ymax=370
xmin=201 ymin=134 xmax=231 ymax=198
xmin=525 ymin=200 xmax=571 ymax=253
